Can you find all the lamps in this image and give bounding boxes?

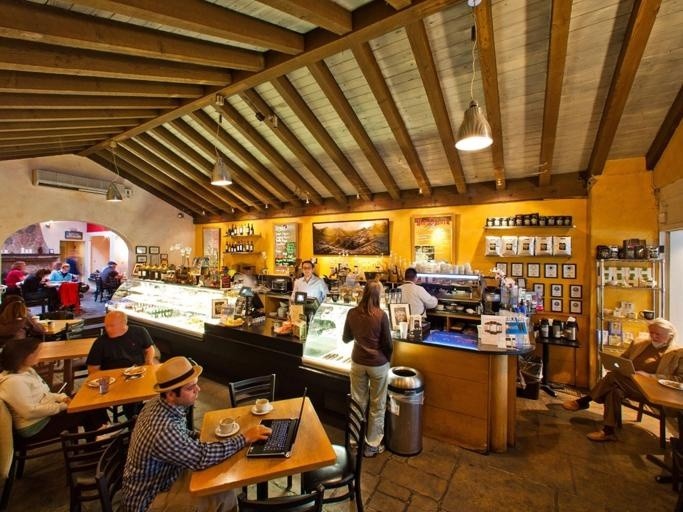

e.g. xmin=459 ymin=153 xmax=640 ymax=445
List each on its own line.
xmin=455 ymin=0 xmax=493 ymax=151
xmin=106 ymin=141 xmax=123 ymax=202
xmin=210 ymin=113 xmax=234 ymax=186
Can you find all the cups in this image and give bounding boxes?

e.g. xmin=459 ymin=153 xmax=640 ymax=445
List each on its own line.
xmin=20 ymin=247 xmax=32 ymax=253
xmin=99 ymin=382 xmax=109 ymax=393
xmin=255 ymin=397 xmax=269 ymax=413
xmin=436 ymin=304 xmax=443 ymax=310
xmin=32 ymin=316 xmax=39 ymax=323
xmin=638 ymin=310 xmax=654 ymax=320
xmin=4 ymin=250 xmax=8 ymax=253
xmin=456 ymin=291 xmax=464 ymax=295
xmin=415 ymin=260 xmax=472 ymax=275
xmin=399 ymin=321 xmax=408 ymax=339
xmin=272 ymin=321 xmax=282 ymax=334
xmin=219 ymin=416 xmax=233 ymax=435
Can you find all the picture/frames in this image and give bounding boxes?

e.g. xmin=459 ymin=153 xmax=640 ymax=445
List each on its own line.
xmin=312 ymin=218 xmax=390 ymax=256
xmin=136 ymin=245 xmax=169 ymax=268
xmin=409 ymin=213 xmax=456 ymax=266
xmin=388 ymin=303 xmax=411 ymax=331
xmin=495 ymin=261 xmax=583 ymax=315
xmin=65 ymin=231 xmax=82 ymax=240
xmin=201 ymin=227 xmax=220 ymax=272
xmin=272 ymin=221 xmax=298 ymax=274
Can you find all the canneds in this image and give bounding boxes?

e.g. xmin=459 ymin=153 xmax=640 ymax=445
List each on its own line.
xmin=487 ymin=215 xmax=570 ymax=226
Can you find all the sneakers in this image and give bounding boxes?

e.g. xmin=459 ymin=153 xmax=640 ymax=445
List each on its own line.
xmin=351 ymin=444 xmax=385 ymax=456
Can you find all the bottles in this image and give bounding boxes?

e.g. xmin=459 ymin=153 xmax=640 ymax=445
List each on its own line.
xmin=137 ymin=268 xmax=166 ymax=281
xmin=552 ymin=320 xmax=565 ymax=339
xmin=229 ymin=223 xmax=254 ymax=236
xmin=512 ymin=305 xmax=517 ymax=312
xmin=328 ymin=267 xmax=336 ymax=280
xmin=330 ymin=287 xmax=339 ymax=303
xmin=610 ymin=246 xmax=618 ymax=259
xmin=47 ymin=321 xmax=54 ymax=332
xmin=566 ymin=316 xmax=578 ymax=342
xmin=124 ymin=303 xmax=173 ymax=320
xmin=391 ymin=270 xmax=397 ymax=282
xmin=224 ymin=240 xmax=254 ymax=253
xmin=540 ymin=318 xmax=548 ymax=338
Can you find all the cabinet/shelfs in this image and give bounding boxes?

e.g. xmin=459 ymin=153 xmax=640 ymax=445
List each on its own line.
xmin=483 ymin=225 xmax=575 ymax=260
xmin=596 ymin=257 xmax=666 ymax=384
xmin=223 ymin=234 xmax=261 ymax=255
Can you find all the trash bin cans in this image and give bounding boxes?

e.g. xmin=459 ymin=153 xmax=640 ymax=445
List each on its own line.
xmin=516 ymin=354 xmax=543 ymax=400
xmin=382 ymin=365 xmax=425 ymax=457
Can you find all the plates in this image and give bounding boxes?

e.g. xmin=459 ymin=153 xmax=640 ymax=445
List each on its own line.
xmin=434 ymin=308 xmax=445 ymax=311
xmin=657 ymin=379 xmax=683 ymax=392
xmin=213 ymin=423 xmax=240 ymax=438
xmin=122 ymin=366 xmax=146 ymax=376
xmin=223 ymin=320 xmax=244 ymax=328
xmin=250 ymin=404 xmax=272 ymax=416
xmin=87 ymin=377 xmax=116 ymax=387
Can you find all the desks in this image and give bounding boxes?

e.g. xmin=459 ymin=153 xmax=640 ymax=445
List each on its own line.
xmin=188 ymin=394 xmax=340 ymax=499
xmin=534 ymin=337 xmax=582 ymax=397
xmin=68 ymin=360 xmax=163 ymax=425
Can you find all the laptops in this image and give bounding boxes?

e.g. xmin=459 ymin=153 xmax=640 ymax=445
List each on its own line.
xmin=597 ymin=352 xmax=636 ymax=378
xmin=244 ymin=384 xmax=307 ymax=459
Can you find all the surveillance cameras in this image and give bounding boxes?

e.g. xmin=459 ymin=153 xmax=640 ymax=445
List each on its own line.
xmin=271 ymin=115 xmax=278 ymax=128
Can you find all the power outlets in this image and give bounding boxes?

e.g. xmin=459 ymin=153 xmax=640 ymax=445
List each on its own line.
xmin=125 ymin=189 xmax=131 ymax=196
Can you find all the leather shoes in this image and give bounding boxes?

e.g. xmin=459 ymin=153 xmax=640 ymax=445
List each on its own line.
xmin=564 ymin=399 xmax=589 ymax=411
xmin=586 ymin=432 xmax=619 ymax=441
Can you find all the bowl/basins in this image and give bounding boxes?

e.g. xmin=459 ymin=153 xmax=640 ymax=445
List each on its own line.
xmin=446 ymin=305 xmax=464 ymax=311
xmin=363 ymin=271 xmax=384 ymax=281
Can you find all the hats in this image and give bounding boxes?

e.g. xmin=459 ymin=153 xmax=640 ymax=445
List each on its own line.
xmin=108 ymin=262 xmax=117 ymax=265
xmin=153 ymin=356 xmax=203 ymax=392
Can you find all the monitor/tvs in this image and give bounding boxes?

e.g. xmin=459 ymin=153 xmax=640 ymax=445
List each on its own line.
xmin=304 ymin=297 xmax=320 ymax=320
xmin=247 ymin=291 xmax=264 ymax=309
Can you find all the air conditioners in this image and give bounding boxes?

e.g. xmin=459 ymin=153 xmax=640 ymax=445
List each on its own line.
xmin=29 ymin=169 xmax=124 ymax=199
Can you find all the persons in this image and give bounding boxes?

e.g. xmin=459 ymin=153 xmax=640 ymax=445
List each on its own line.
xmin=0 ymin=336 xmax=122 ymax=447
xmin=290 ymin=260 xmax=329 ymax=302
xmin=342 ymin=281 xmax=394 ymax=457
xmin=561 ymin=317 xmax=683 ymax=441
xmin=85 ymin=309 xmax=155 ymax=440
xmin=120 ymin=356 xmax=273 ymax=512
xmin=396 ymin=267 xmax=438 ymax=318
xmin=0 ymin=255 xmax=122 ymax=343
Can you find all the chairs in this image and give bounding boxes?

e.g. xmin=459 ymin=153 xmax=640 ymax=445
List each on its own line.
xmin=185 ymin=356 xmax=200 ymax=431
xmin=301 ymin=393 xmax=370 ymax=511
xmin=1 ymin=272 xmax=126 ymax=389
xmin=229 ymin=372 xmax=278 ymax=409
xmin=1 ymin=400 xmax=79 ymax=484
xmin=620 ymin=359 xmax=683 ymax=449
xmin=61 ymin=404 xmax=139 ymax=512
xmin=236 ymin=489 xmax=324 ymax=511
